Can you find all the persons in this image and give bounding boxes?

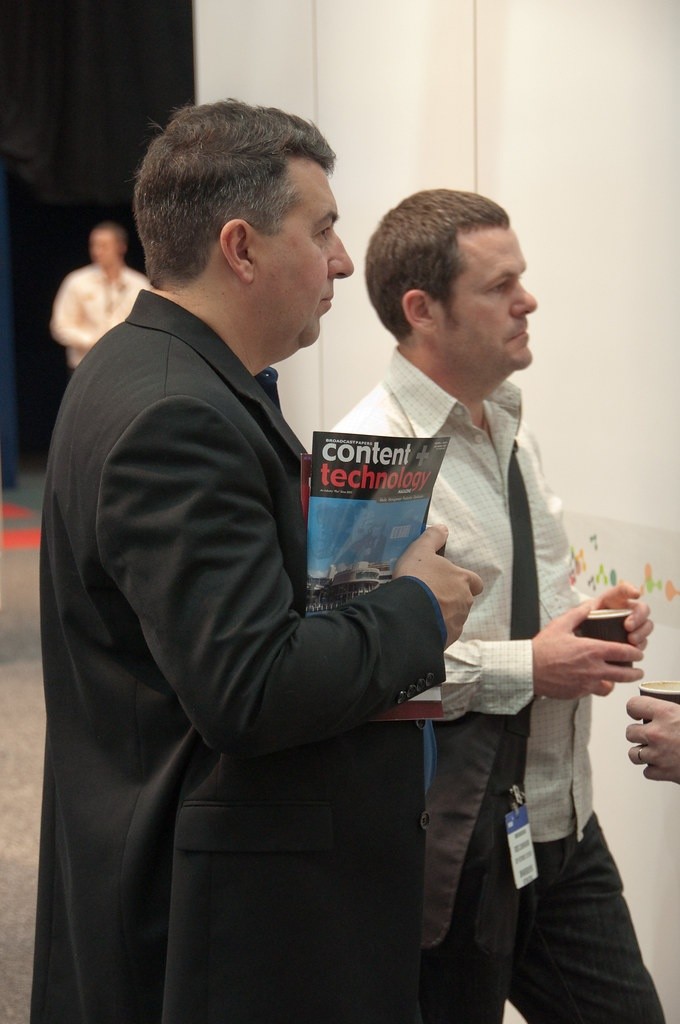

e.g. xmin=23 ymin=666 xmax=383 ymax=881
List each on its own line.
xmin=627 ymin=696 xmax=680 ymax=781
xmin=50 ymin=224 xmax=150 ymax=368
xmin=328 ymin=189 xmax=668 ymax=1023
xmin=32 ymin=100 xmax=483 ymax=1024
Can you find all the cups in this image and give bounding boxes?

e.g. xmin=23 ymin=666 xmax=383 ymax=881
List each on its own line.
xmin=638 ymin=682 xmax=680 ymax=724
xmin=580 ymin=608 xmax=633 ymax=669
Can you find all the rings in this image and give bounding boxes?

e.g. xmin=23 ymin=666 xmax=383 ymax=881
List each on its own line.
xmin=638 ymin=746 xmax=645 ymax=763
